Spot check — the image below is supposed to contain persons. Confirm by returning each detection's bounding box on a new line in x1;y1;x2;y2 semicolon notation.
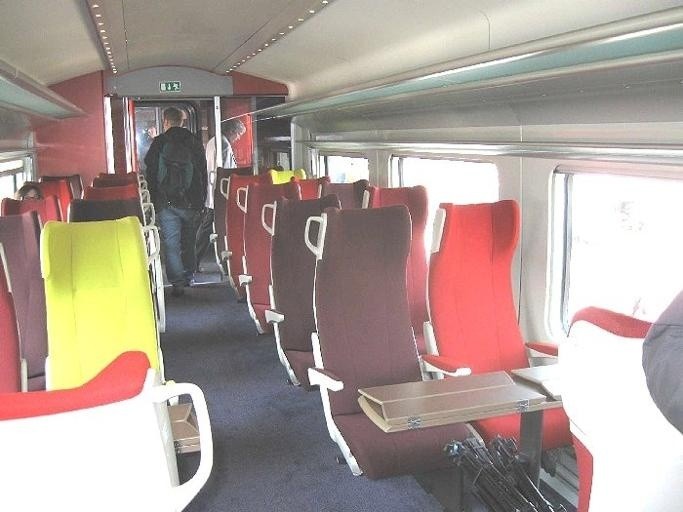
142;106;210;298
12;183;41;201
192;117;247;276
641;289;681;438
141;126;158;148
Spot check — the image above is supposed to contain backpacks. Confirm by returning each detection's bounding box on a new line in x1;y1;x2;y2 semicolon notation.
155;131;196;198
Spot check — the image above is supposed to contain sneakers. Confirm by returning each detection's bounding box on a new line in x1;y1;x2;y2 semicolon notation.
184;278;196;288
170;280;185;298
